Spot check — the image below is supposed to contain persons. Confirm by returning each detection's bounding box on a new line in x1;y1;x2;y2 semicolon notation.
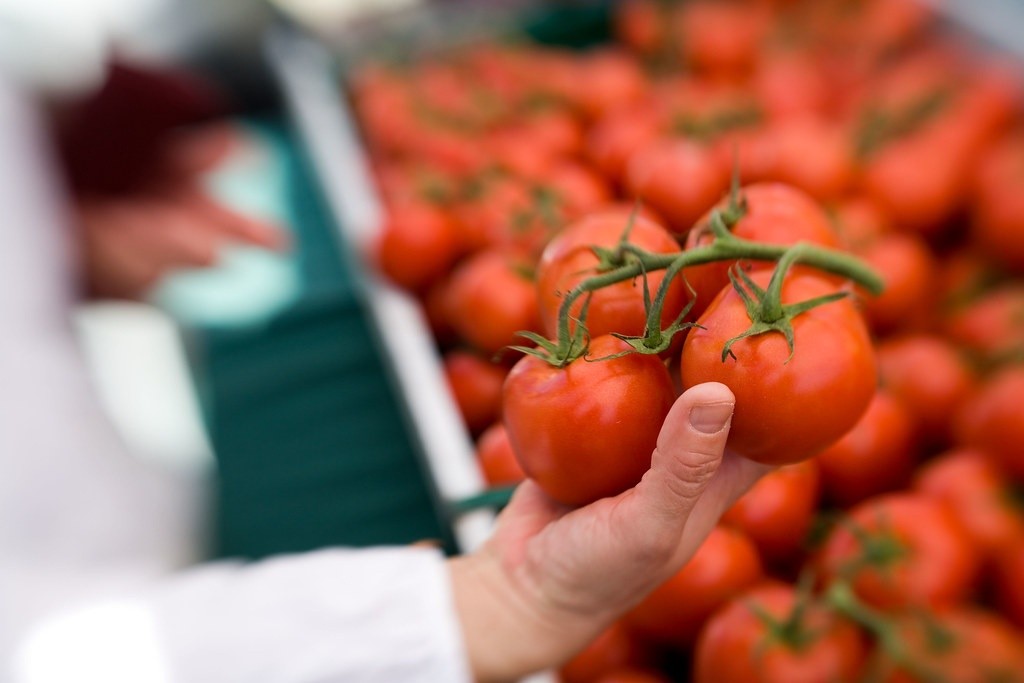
1;1;782;683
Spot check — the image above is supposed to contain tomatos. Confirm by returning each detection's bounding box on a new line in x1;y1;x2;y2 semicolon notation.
352;0;1024;683
